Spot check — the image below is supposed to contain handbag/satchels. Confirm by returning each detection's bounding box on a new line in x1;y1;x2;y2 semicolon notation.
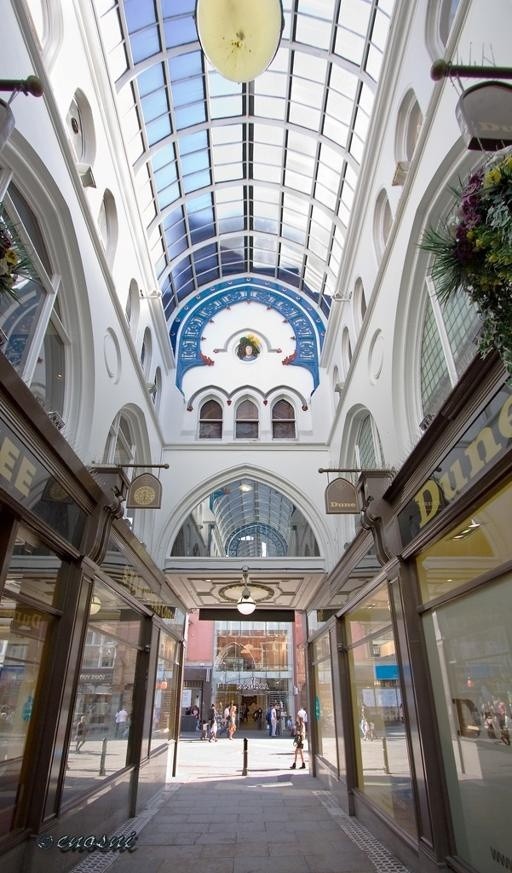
74;736;82;742
226;716;230;721
294;736;301;744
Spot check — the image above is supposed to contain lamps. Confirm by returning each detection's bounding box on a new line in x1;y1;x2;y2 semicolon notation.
236;567;256;616
195;0;283;85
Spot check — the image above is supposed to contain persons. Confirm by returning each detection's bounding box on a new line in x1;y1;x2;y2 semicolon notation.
359;702;375;741
267;702;281;736
290;716;306;770
493;695;511;745
115;706;129;739
200;702;238;743
185;705;199;731
74;715;88;754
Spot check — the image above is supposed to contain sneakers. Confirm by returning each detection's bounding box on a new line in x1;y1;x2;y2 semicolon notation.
299;764;306;769
290;763;296;769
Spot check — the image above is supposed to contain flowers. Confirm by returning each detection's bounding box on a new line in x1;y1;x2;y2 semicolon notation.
407;140;512;392
2;204;41;311
